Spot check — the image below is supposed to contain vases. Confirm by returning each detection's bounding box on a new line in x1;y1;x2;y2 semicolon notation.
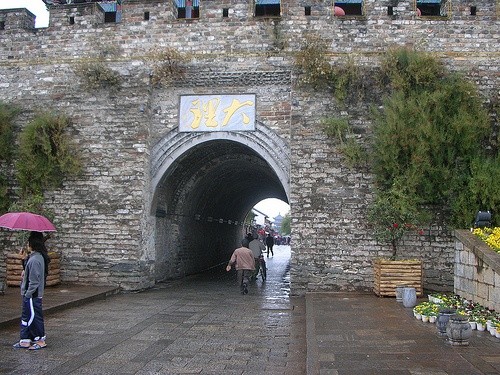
372;260;424;298
469;322;476;330
477;323;486;331
428;316;436;323
422;314;428;322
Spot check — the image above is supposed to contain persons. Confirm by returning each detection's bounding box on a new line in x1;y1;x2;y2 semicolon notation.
13;236;47;349
22;231;49;344
228;232;291;294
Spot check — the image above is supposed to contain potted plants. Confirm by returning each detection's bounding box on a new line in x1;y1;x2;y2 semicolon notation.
6;195;61;288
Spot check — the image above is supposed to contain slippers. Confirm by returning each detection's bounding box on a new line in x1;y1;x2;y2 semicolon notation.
28;343;47;350
13;342;32;348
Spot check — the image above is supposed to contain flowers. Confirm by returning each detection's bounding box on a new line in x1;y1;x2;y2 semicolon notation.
413;290;500;333
369;175;432;262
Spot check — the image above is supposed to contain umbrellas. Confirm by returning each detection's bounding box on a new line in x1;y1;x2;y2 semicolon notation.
0;212;57;248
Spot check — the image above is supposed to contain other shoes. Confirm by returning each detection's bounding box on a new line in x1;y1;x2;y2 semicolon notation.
241;291;246;295
243;285;248;293
